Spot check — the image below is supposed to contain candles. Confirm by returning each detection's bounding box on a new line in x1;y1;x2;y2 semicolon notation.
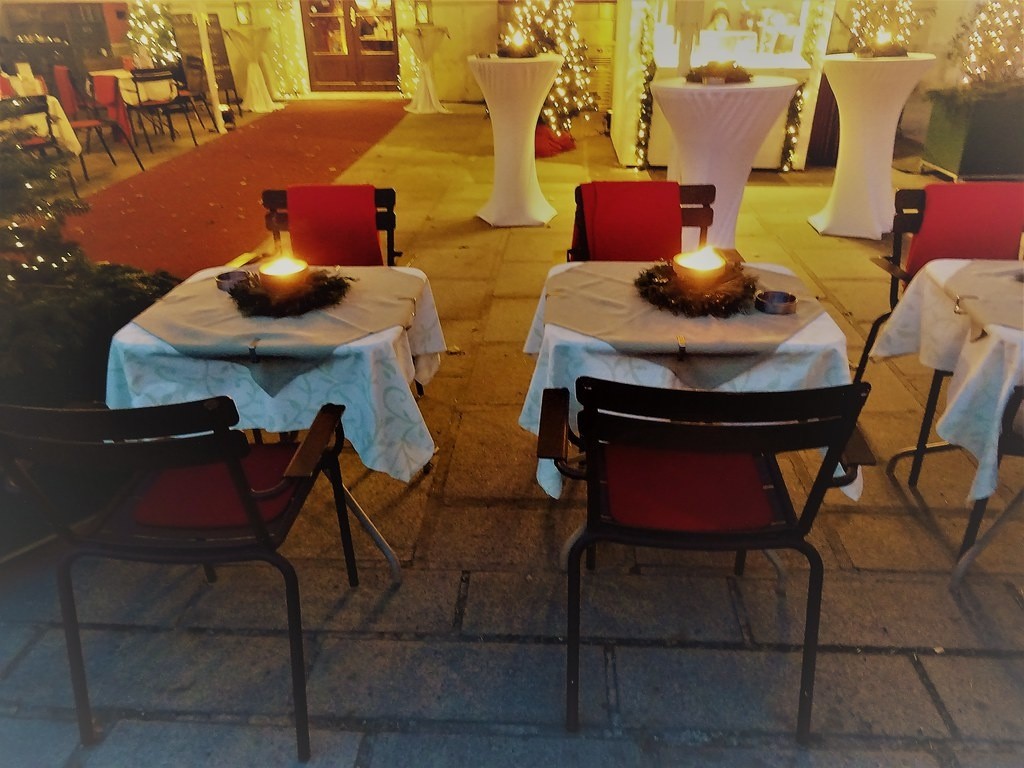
259;256;307;291
674;245;726;283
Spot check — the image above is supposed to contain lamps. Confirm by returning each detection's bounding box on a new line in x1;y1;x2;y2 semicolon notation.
235;1;251;26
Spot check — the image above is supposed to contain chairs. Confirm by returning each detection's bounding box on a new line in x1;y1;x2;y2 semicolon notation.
0;57;244;198
0;179;1024;745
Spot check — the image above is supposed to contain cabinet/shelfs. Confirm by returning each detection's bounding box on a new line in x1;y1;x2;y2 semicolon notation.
921;92;1024;183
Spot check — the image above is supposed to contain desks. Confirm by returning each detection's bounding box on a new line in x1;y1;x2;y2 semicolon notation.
867;258;1024;563
415;1;434;26
1;95;82;156
106;266;449;587
1;76;43;100
224;25;285;113
517;262;862;575
651;76;800;249
397;25;455;113
806;49;936;240
85;69;180;138
467;56;562;226
